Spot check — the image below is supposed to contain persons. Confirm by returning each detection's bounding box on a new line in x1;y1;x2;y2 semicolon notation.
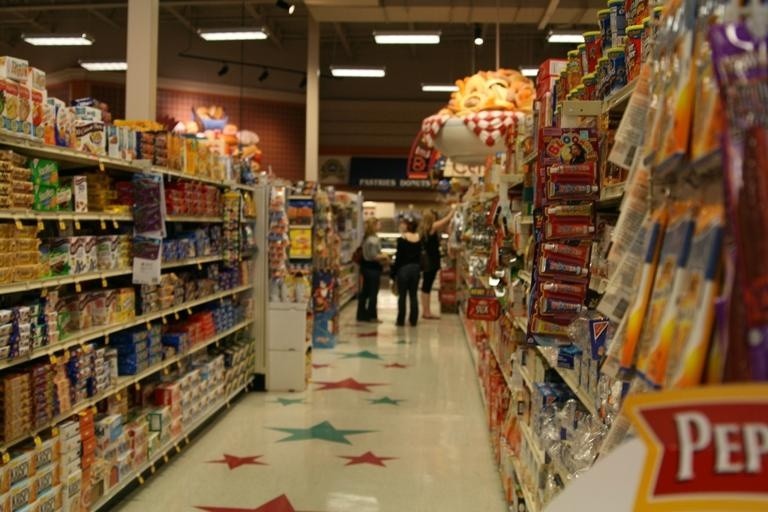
421;205;456;320
357;217;390;322
391;212;421;327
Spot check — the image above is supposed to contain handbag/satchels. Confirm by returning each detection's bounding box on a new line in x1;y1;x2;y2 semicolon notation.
354;235;366;262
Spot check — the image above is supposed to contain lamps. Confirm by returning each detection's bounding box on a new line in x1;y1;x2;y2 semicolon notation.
544;28;586;47
77;52;129;75
418;79;457;94
195;16;270;43
20;13;98;47
519;64;541;78
472;27;485;47
328;56;388;82
276;4;298;19
373;27;442;50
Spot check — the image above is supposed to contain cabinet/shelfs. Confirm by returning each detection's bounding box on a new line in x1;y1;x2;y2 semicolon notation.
0;131;269;512
